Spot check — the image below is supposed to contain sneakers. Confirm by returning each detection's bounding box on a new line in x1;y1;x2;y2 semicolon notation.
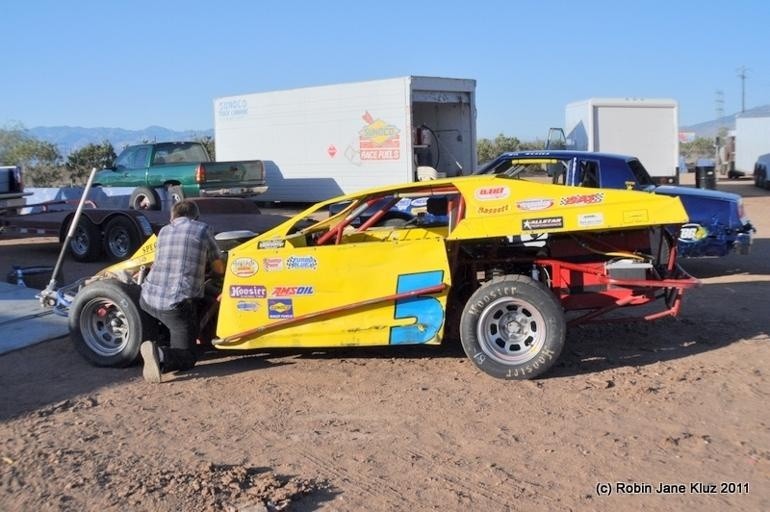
140;340;162;384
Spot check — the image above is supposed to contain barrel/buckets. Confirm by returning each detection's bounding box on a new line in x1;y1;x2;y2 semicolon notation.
695;166;717;190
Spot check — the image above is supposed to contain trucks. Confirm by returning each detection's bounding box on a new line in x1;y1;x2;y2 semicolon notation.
546;97;679;188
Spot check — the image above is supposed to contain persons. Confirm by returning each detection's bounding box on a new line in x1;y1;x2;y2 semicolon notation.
137;199;224;383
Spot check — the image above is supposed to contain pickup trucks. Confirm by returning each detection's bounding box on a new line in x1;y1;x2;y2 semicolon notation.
91;142;269;201
